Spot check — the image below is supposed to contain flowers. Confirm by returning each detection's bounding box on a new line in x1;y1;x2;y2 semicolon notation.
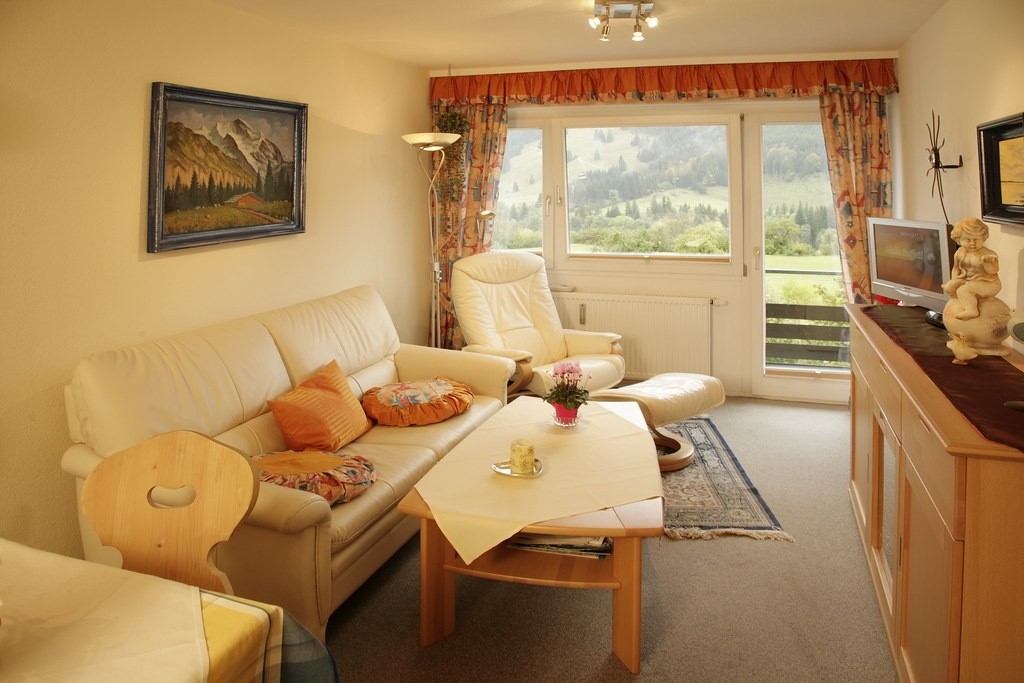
544;360;594;410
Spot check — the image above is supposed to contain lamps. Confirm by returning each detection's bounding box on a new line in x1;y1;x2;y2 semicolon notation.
432;210;496;255
589;0;658;42
402;132;461;350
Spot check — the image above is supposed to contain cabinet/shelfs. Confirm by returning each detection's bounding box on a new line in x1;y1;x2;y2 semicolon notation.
841;300;1024;683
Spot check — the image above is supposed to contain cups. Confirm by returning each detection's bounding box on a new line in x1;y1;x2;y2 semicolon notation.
510;438;534;474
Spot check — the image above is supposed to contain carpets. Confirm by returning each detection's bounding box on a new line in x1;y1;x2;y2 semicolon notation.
647;412;799;546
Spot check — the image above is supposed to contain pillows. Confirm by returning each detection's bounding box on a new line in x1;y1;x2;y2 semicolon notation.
362;375;473;428
248;446;375;510
266;359;375;453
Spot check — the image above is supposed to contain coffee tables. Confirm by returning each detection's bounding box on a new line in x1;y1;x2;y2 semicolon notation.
401;395;665;673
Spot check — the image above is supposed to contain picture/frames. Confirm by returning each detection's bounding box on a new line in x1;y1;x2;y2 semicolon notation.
144;81;309;252
975;111;1024;229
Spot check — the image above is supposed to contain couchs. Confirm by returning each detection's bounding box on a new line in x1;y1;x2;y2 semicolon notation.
60;283;519;651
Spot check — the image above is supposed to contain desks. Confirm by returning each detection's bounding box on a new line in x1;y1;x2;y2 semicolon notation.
0;535;337;683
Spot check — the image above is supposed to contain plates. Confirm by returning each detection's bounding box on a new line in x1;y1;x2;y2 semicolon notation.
491;458;544;478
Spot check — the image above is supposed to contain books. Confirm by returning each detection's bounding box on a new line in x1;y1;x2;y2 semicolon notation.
507;532;612;560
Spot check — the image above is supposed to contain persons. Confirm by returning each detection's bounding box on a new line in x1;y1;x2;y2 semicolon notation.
941;216;1002;320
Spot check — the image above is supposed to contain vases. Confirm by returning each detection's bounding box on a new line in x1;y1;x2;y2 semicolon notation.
548;401;583;426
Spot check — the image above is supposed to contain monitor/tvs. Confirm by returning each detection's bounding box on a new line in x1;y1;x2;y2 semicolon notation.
867;217;959;314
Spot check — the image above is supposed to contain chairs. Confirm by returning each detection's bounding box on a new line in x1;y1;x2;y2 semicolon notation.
75;429;262;597
449;249;725;473
